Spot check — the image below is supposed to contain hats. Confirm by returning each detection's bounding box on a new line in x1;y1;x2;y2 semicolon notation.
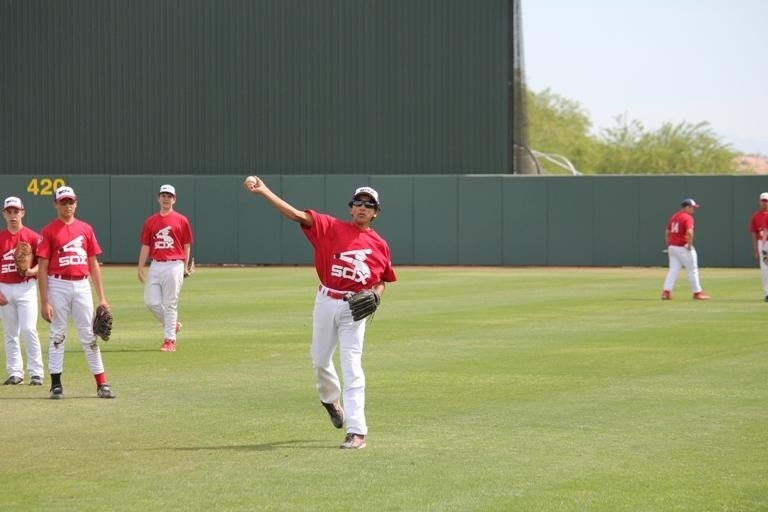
56;186;76;201
4;197;24;210
679;199;700;208
353;187;380;205
760;193;768;201
158;185;176;196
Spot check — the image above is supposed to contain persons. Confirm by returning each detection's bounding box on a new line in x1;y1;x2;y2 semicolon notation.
39;185;118;399
749;191;768;304
0;195;44;388
242;174;397;451
136;183;194;351
655;197;710;301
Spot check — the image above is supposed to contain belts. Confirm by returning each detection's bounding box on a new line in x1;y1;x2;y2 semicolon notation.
49;273;90;280
319;285;347;299
150;258;186;262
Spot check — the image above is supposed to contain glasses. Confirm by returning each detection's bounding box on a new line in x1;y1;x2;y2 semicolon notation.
352;199;376;208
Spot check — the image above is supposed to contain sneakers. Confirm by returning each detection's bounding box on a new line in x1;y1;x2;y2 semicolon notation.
320;399;345;428
693;293;710;299
160;339;176;352
30;375;43;384
50;385;64;399
176;322;181;333
340;433;366;449
3;376;23;385
97;384;115;398
661;291;670;300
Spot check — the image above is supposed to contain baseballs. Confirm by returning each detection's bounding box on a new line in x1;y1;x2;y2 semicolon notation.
246;177;256;186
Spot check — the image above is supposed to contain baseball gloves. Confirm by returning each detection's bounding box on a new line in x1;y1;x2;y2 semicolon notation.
13;239;33;277
94;306;113;342
184;256;195;278
344;289;380;321
762;251;768;265
